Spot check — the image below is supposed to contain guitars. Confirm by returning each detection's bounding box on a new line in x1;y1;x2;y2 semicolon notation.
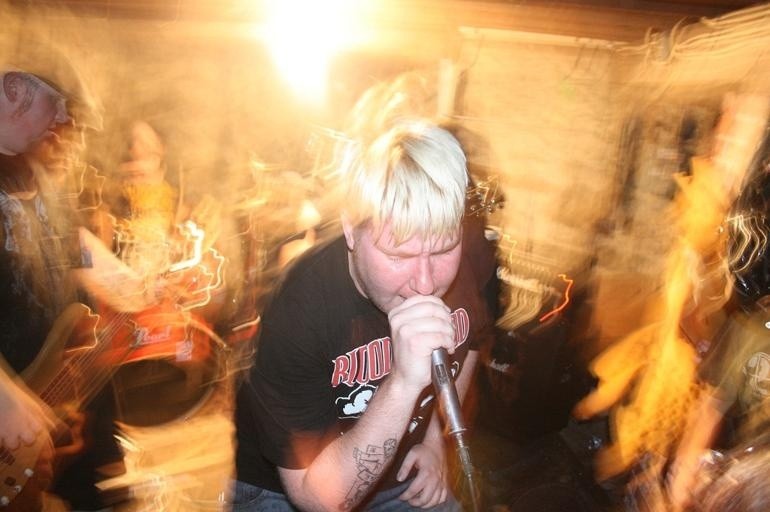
1;251;196;510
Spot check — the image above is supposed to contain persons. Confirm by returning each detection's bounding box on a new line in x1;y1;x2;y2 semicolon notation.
1;72;152;510
228;123;497;511
659;127;769;511
131;120;173;280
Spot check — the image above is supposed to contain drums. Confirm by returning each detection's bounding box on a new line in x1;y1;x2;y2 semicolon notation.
86;301;217;428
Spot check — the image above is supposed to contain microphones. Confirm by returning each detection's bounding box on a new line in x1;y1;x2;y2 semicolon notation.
431;349;469;454
